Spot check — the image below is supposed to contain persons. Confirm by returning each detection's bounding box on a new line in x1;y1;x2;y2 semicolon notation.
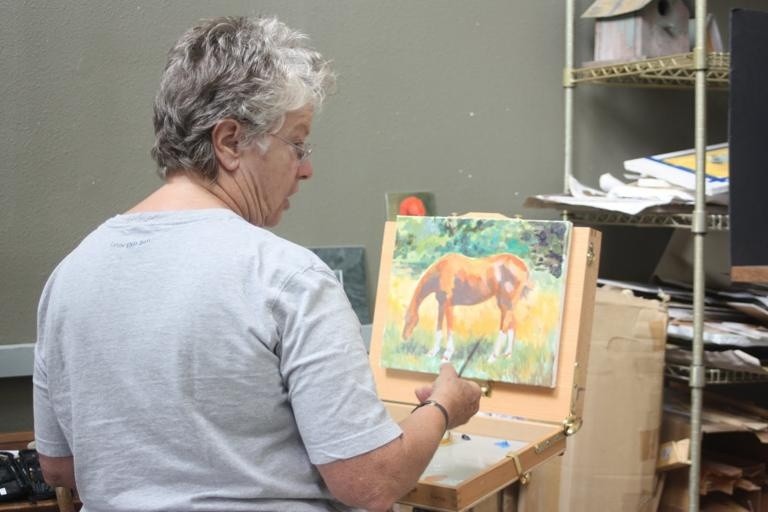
31;10;480;512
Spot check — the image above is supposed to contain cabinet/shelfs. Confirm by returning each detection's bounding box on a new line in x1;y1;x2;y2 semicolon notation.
561;0;766;512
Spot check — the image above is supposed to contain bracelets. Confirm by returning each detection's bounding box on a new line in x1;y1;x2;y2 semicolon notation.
408;400;451;433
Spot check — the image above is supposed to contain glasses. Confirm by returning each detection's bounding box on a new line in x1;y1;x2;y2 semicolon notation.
262;125;314;164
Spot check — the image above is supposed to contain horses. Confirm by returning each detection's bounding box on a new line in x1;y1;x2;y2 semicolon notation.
400;252;535;364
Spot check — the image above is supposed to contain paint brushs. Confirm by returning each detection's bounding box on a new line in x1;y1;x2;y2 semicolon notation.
457;333;485;379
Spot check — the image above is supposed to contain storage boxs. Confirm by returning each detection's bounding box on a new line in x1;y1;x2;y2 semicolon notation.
468;288;673;512
